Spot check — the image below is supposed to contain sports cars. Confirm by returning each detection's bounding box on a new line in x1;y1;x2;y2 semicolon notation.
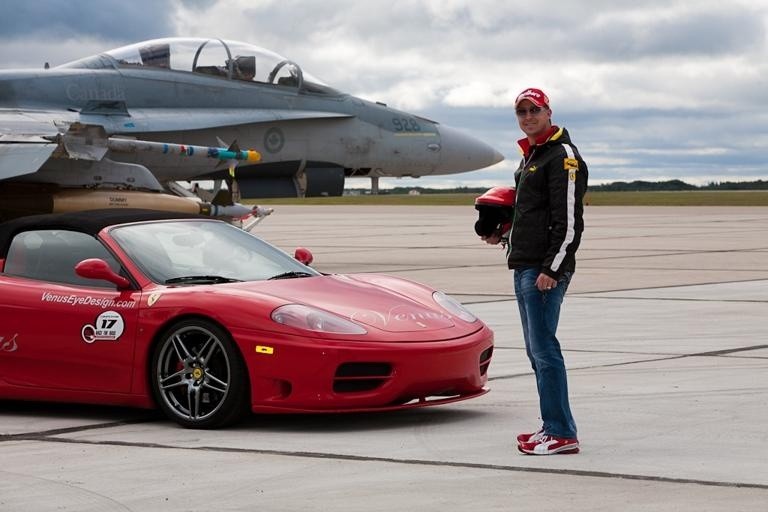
0;219;495;430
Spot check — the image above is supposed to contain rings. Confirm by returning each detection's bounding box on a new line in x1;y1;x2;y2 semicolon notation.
547;287;552;289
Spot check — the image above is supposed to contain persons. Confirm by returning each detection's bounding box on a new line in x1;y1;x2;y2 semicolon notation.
481;87;588;456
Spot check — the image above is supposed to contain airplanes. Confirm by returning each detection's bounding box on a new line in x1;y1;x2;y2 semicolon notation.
0;36;508;231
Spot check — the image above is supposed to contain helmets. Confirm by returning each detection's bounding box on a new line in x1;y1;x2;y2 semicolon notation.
474;186;516;238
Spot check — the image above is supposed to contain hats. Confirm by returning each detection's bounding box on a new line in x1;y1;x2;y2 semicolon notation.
515;88;549;109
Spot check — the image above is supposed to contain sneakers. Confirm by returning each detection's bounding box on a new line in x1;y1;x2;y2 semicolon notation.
517;427;580;455
